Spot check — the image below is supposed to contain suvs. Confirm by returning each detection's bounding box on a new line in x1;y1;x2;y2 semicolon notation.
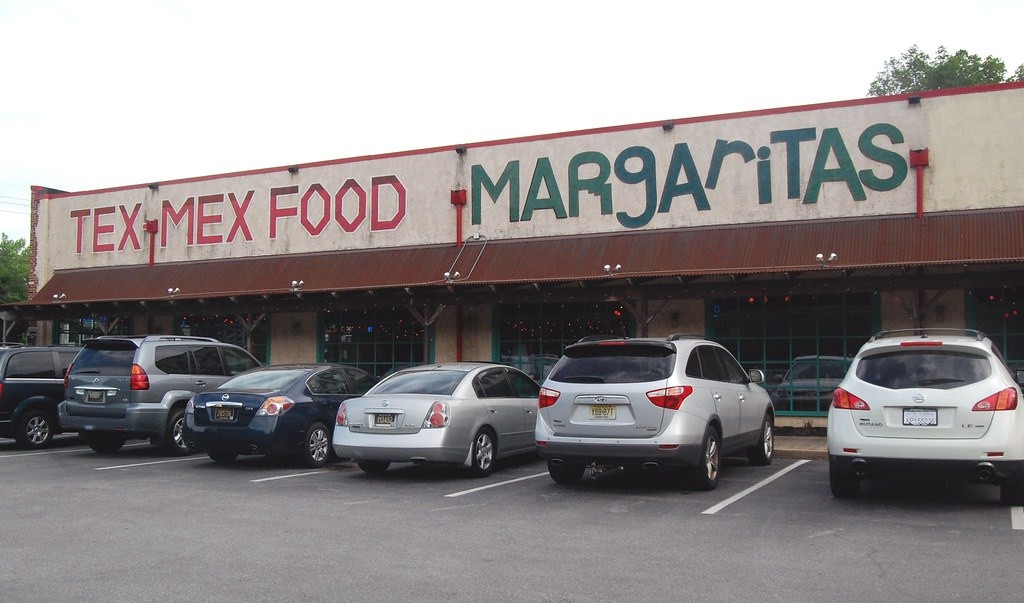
771;354;853;415
534;332;776;490
0;342;83;451
56;335;264;457
501;354;560;383
826;326;1023;499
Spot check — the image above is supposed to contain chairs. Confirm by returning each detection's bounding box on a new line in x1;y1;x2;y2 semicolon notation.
877;361;909;389
316;375;340;394
950;359;974;380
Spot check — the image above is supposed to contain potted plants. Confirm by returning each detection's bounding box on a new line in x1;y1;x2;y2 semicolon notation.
51;294;66;302
289;280;305;290
167;288;181;297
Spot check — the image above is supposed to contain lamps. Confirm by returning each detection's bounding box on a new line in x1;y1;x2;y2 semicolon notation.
148;184;159;190
934;305;945;322
815;252;837;269
443;272;460;282
287;168;298;173
663;123;674;129
455;147;467;153
604;264;622;278
907;95;922;104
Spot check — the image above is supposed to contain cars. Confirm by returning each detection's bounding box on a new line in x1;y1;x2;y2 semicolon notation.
332;363;540;477
182;364;379;468
